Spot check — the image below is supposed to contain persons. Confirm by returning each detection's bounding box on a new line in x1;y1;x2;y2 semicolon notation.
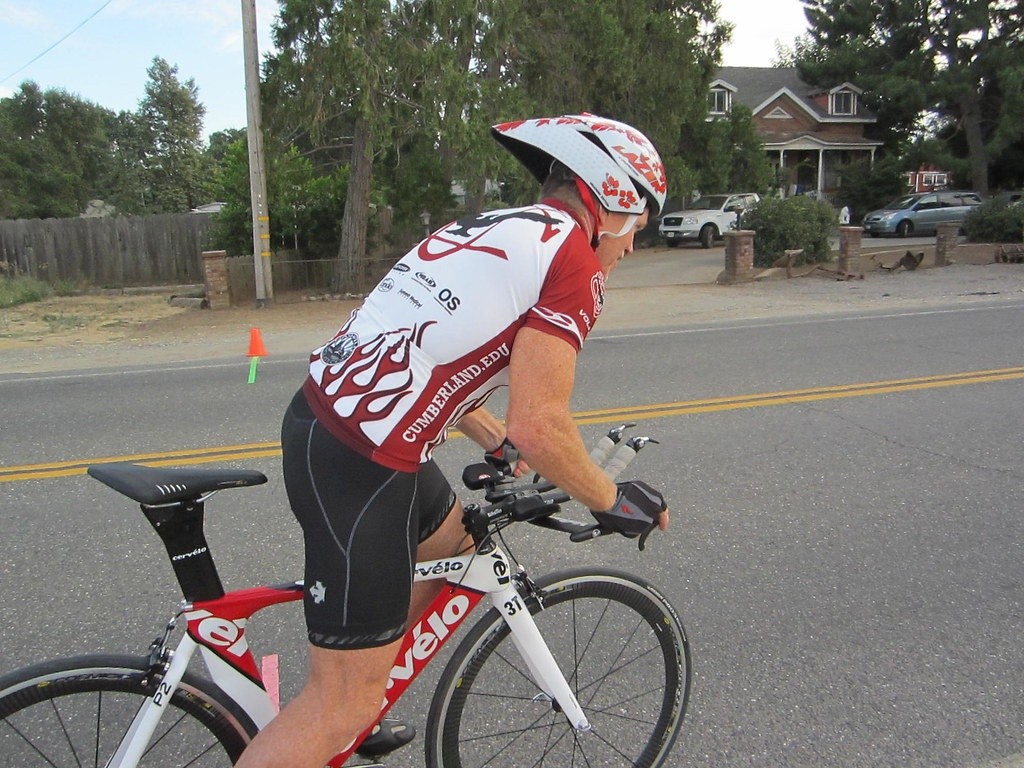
231;112;673;768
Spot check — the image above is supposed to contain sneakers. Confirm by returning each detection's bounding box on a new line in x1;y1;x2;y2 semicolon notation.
353;718;415;756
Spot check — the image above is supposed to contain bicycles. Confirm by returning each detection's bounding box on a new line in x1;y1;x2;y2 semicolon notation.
0;422;692;768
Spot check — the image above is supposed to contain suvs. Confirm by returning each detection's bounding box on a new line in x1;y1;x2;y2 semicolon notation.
659;192;760;249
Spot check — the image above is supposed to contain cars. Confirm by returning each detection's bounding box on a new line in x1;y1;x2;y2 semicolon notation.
864;191;984;238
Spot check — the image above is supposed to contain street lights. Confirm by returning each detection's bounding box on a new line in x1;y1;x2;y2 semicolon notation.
419;207;430;238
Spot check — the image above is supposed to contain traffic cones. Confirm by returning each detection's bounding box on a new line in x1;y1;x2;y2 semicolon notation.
246;327;267;357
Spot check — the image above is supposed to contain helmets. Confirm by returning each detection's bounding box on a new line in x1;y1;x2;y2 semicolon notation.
491;110;667;219
